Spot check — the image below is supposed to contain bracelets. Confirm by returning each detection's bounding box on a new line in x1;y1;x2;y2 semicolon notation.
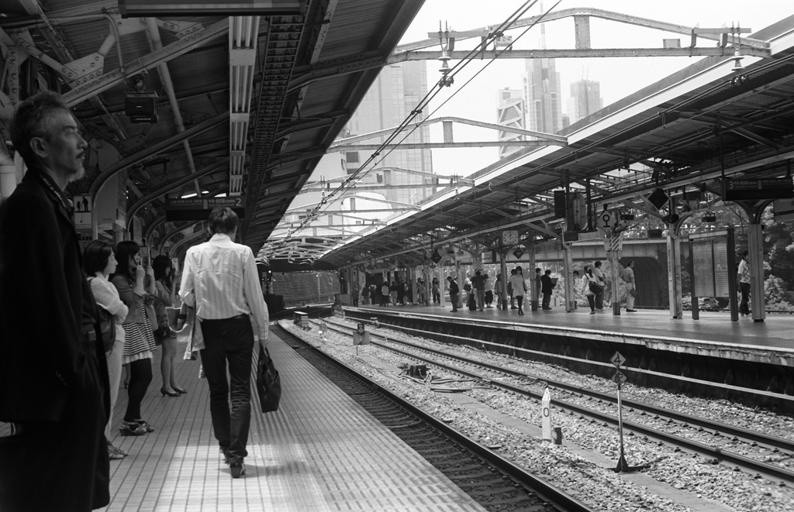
176;312;186;320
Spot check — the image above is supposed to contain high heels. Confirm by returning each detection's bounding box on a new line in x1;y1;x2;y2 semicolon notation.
161;387;180;397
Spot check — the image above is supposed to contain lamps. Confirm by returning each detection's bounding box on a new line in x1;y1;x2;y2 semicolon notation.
228;15;260;197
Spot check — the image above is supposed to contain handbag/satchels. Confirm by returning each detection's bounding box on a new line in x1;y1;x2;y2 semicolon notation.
153;324;170;341
256;339;281;413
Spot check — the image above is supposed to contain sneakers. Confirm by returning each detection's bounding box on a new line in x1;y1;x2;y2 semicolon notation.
107;440;128;458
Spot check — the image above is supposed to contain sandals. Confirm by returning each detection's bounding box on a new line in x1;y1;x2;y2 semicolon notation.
119;421;147;436
135;419;154;433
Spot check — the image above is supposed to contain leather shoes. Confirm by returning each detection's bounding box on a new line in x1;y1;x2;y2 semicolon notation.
171;384;186;393
232;463;246;478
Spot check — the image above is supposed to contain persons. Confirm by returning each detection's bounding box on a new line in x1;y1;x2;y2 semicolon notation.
80;239;127;460
0;90;110;512
360;258;637;316
738;250;751;315
176;206;269;478
108;241;157;435
146;255;187;397
